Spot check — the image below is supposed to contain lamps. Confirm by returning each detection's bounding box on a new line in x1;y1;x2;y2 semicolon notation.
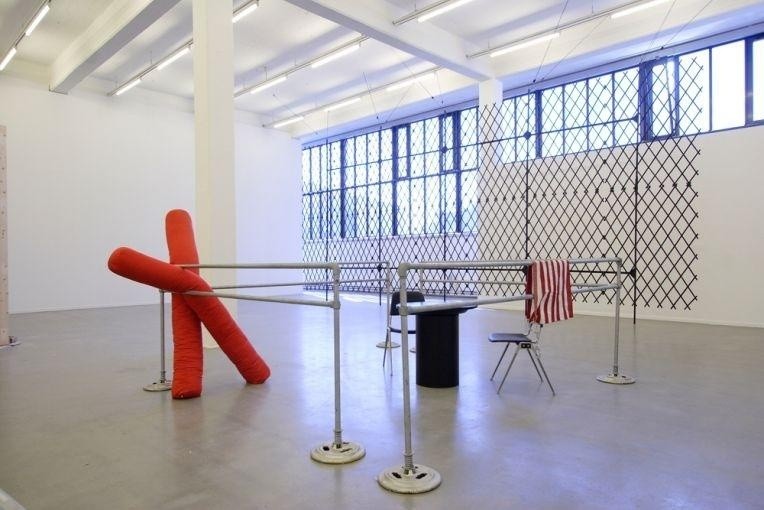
462;0;672;60
0;0;52;72
233;0;259;23
112;39;193;95
262;66;445;128
231;37;369;98
392;0;478;27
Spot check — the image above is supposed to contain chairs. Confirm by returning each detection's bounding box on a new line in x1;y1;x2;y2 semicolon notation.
383;290;425;376
488;322;555;397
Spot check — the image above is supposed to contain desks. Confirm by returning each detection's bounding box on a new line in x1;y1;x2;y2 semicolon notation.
410;307;478;389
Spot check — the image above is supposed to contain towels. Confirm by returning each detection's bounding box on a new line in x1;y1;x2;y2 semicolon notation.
523;259;574;323
